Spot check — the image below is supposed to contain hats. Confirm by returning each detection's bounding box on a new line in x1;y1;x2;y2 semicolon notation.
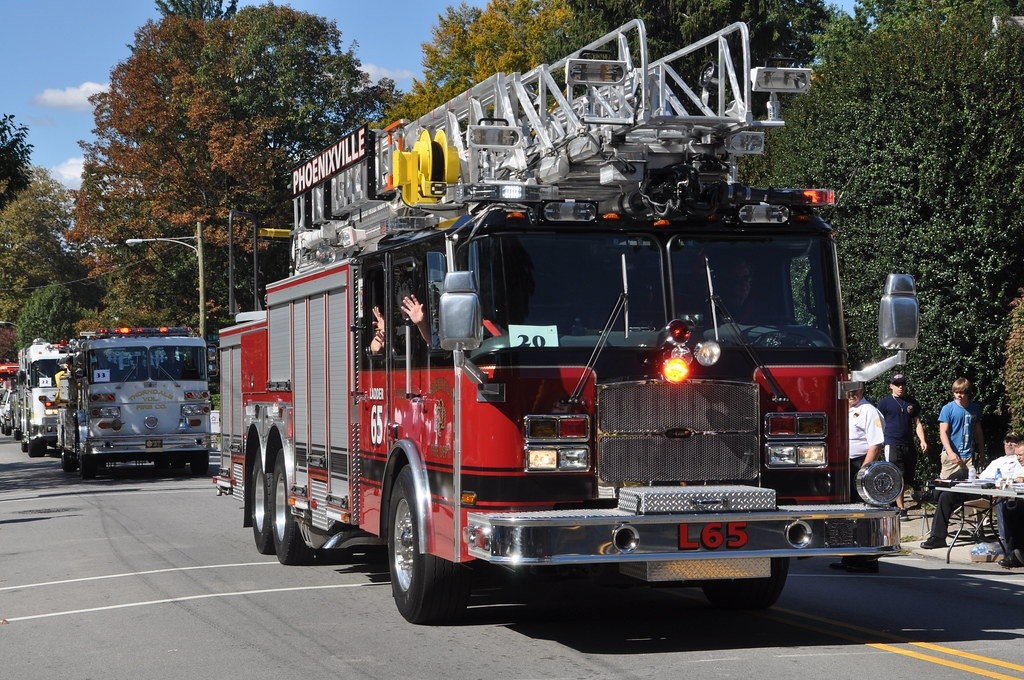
1005;431;1019;441
891;374;905;384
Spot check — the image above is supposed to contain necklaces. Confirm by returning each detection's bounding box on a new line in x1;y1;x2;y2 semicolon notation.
893;396;905;412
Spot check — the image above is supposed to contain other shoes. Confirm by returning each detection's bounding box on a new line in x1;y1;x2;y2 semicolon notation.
999;549;1024;566
911;489;934;503
921;537;946;548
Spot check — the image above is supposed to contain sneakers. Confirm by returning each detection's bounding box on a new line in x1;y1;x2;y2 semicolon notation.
899;508;908;521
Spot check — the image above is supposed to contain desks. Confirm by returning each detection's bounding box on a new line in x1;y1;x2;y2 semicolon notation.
936;487;1024;566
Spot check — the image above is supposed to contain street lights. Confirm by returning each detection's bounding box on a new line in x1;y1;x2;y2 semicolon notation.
124;221;206;340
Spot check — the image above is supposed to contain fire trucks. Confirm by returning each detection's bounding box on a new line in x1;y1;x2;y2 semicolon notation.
209;15;921;630
0;326;218;479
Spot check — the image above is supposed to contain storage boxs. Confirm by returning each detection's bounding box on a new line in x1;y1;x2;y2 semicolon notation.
971;554;992;562
991;554;1005;562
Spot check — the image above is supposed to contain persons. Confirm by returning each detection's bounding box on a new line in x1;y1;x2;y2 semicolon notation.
829;381;886;573
940;378;995;528
920;432;1024;568
369;282;414;356
400;294;431;344
877;374;928;522
